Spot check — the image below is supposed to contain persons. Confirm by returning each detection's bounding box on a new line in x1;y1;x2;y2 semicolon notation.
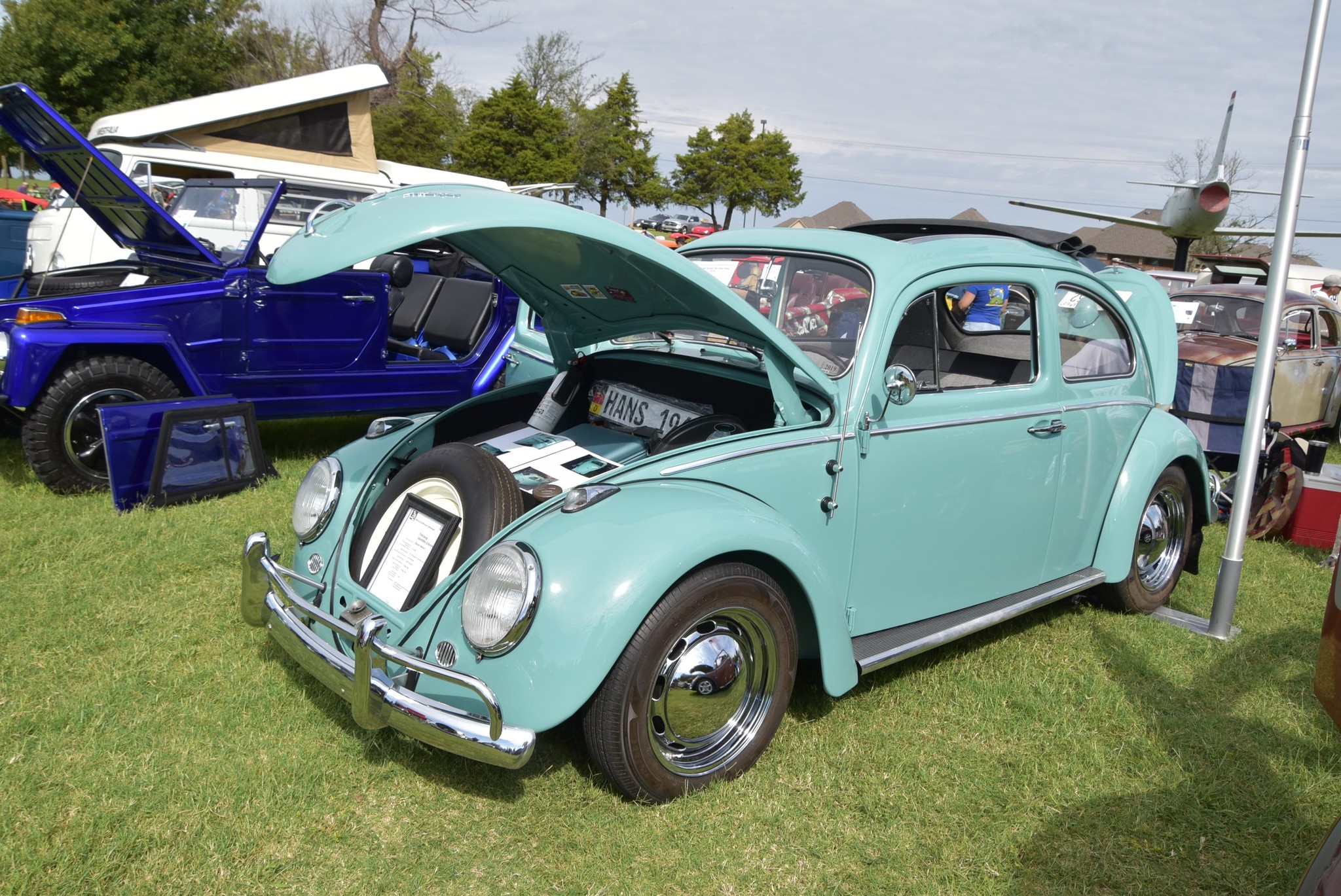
28;183;39;192
958;283;1010;332
1310;274;1341;299
17;182;29;194
642;231;647;236
676;236;685;247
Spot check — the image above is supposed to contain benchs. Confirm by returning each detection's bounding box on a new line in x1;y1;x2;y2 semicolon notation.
1238;331;1335;347
885;342;1103;385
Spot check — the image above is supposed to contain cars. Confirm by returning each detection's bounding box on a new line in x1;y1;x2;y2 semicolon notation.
237;185;1222;807
1168;283;1341;456
1144;254;1341;320
633;215;871;339
0;81;519;512
128;177;378;280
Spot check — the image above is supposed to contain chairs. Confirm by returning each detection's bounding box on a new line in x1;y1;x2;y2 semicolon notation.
786;272;814;307
815;274;854;302
801;350;853;376
1294;344;1312;350
386;278;494;363
910;368;1011;389
343;253;413;320
387;271;447;342
786;280;816;307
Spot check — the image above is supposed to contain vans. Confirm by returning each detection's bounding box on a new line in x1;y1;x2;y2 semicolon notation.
23;143;393;276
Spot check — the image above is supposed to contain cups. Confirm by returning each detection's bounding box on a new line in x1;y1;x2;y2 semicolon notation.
1304;440;1329;476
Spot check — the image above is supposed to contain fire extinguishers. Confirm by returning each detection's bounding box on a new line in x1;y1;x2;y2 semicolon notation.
528;351;586;433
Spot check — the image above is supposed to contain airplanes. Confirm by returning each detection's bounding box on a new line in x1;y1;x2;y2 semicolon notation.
1008;90;1341;246
